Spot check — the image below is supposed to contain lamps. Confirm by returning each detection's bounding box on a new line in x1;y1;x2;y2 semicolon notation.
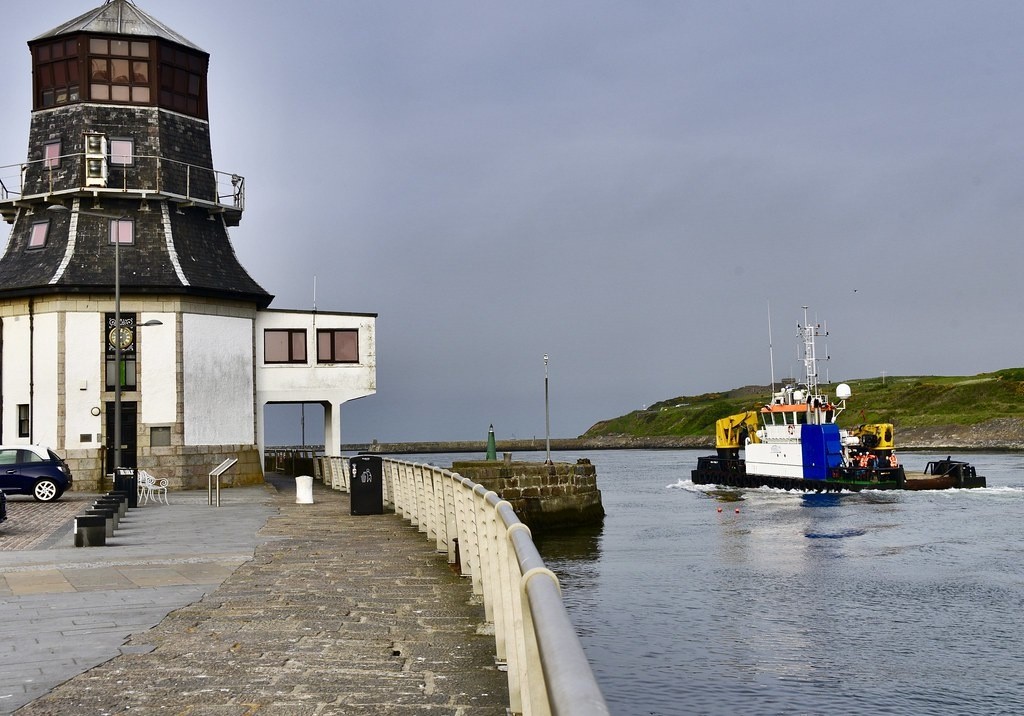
175;208;184;215
138;202;152;212
90;202;104;210
25;209;34;216
207;215;215;221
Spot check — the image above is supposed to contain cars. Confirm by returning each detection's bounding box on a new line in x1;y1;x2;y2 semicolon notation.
0;443;74;503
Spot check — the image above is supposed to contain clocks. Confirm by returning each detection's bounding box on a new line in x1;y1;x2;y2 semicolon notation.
111;328;131;346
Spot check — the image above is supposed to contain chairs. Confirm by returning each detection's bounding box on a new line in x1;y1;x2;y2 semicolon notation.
138;470;169;505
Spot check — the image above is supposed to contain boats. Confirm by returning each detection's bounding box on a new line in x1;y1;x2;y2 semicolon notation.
689;298;988;496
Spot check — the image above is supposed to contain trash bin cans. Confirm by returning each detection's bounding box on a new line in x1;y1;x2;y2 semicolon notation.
351;456;382;514
113;469;138;508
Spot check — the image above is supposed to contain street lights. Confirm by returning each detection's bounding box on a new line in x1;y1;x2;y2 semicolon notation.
46;203;164;485
